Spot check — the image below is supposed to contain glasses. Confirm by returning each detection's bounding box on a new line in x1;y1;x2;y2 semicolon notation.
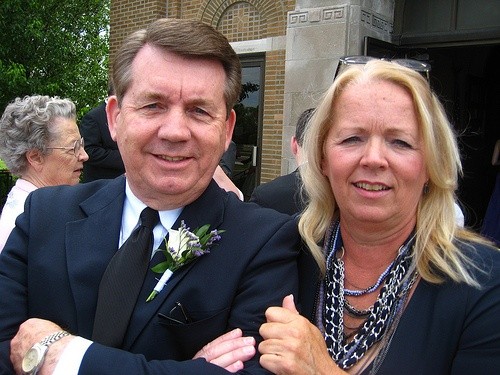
46;137;84;158
334;55;431;84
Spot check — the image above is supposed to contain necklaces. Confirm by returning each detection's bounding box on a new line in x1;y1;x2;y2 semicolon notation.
310;207;421;375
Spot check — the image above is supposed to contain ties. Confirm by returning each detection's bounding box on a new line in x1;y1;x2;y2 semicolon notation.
93;206;160;349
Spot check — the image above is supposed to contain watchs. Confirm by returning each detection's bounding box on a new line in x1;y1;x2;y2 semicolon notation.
21;331;71;374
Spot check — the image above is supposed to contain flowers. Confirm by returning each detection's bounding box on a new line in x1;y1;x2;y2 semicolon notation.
146;220;227;301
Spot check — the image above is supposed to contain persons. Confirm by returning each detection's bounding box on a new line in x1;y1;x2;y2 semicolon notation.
78;86;126;183
258;56;500;375
1;94;89;255
0;18;301;375
249;108;315;216
